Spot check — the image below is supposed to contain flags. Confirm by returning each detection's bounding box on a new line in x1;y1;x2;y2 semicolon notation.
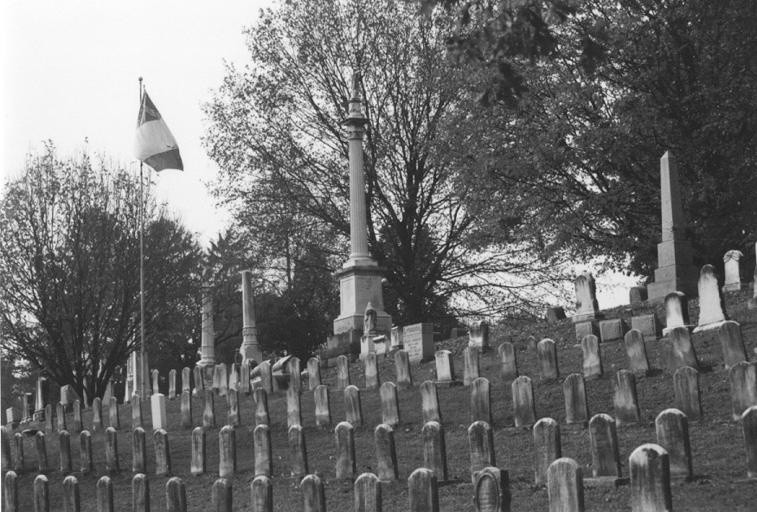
133;87;184;172
